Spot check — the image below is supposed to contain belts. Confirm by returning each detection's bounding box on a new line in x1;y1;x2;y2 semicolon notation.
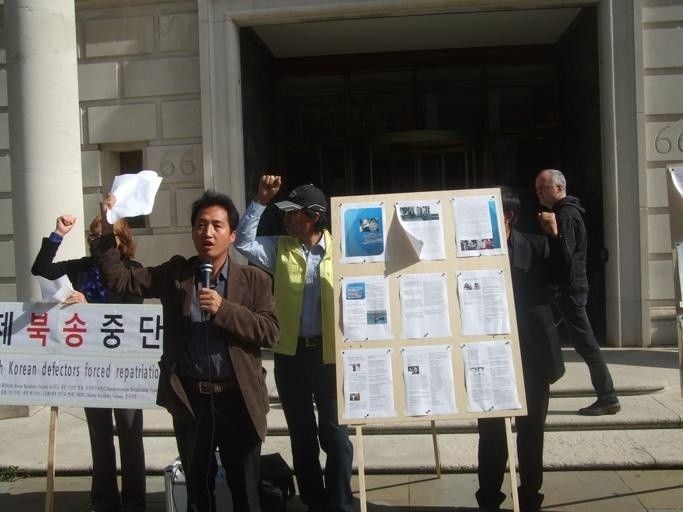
194;382;233;394
299;336;322;347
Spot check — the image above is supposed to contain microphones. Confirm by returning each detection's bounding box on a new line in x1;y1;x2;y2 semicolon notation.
198;255;213;316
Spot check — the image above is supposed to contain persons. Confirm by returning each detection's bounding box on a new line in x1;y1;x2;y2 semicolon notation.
535;168;621;417
474;185;575;512
232;173;354;512
30;213;146;511
88;188;282;512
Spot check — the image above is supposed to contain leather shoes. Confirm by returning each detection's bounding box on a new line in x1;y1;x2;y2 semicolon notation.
579;400;621;416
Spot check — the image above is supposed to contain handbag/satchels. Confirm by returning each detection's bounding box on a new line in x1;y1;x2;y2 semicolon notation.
258;453;295;512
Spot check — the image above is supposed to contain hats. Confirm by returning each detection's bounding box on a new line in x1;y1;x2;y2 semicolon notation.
274;184;327;212
